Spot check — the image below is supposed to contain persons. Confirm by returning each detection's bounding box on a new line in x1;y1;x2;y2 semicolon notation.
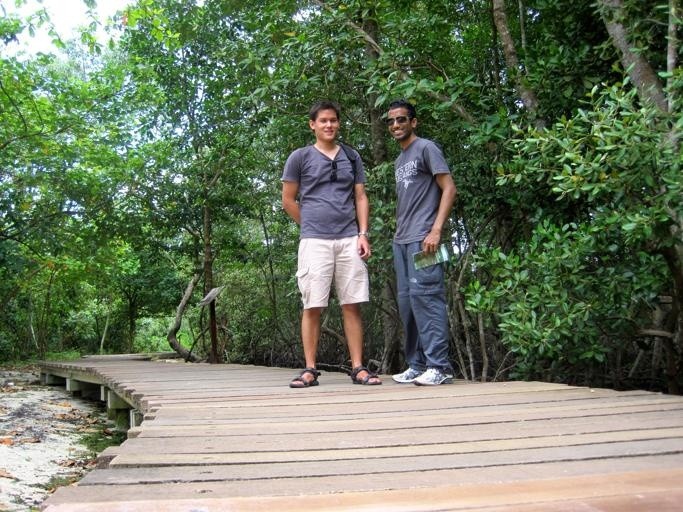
386;99;457;386
279;101;381;388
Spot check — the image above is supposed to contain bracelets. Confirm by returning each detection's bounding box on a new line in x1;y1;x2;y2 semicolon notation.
359;232;369;237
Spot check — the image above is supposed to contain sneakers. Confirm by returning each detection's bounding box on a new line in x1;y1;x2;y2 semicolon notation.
391;367;423;383
414;368;454;385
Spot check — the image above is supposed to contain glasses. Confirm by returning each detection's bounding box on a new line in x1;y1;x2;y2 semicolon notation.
330;160;338;182
386;116;410;125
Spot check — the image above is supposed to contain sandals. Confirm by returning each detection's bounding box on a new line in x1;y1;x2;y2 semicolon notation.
289;368;318;387
350;367;381;384
